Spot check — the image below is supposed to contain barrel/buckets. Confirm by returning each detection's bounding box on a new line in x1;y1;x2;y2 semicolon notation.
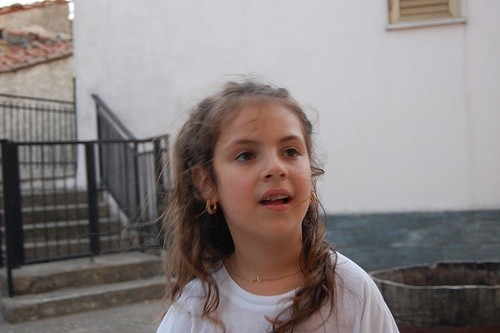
366;259;500;333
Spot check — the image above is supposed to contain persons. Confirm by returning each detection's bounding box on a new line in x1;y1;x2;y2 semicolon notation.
155;80;399;333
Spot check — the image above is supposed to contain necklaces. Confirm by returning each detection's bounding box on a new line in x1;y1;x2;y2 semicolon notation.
227;254;306;283
